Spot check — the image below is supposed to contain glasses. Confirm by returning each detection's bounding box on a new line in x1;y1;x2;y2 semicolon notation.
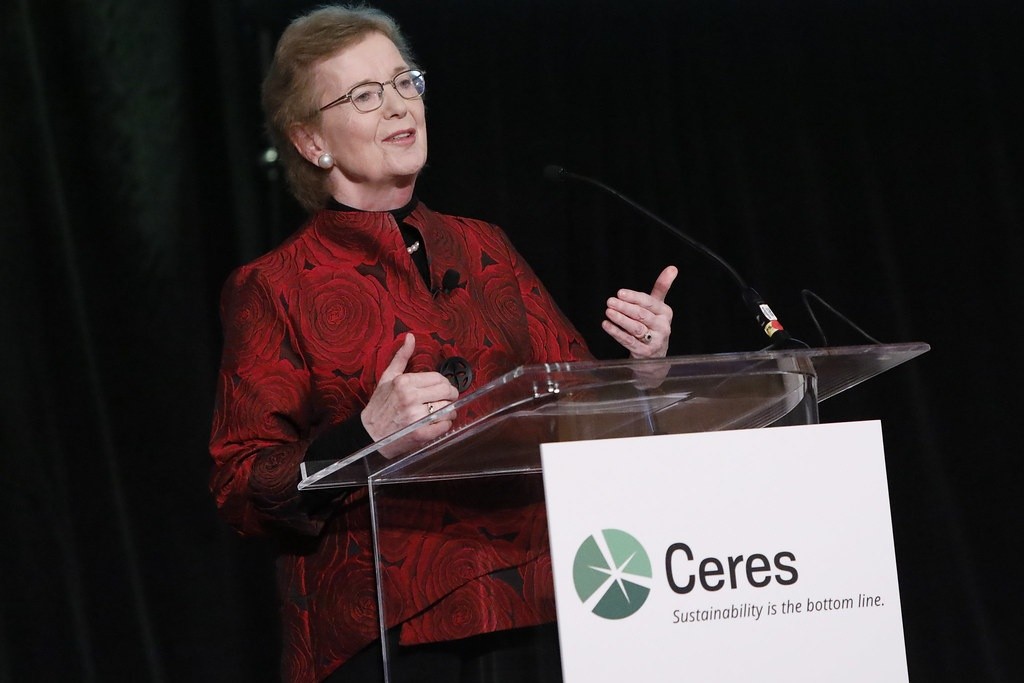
303;69;427;118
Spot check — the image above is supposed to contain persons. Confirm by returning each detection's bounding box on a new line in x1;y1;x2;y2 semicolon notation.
211;4;679;683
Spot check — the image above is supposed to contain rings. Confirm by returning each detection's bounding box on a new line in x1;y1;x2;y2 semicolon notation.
640;329;653;343
427;402;434;414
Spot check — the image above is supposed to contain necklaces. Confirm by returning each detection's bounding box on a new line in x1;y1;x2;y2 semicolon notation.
407;240;420;254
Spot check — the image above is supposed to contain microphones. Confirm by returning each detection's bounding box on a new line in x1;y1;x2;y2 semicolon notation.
431;269;460;300
544;164;808;350
